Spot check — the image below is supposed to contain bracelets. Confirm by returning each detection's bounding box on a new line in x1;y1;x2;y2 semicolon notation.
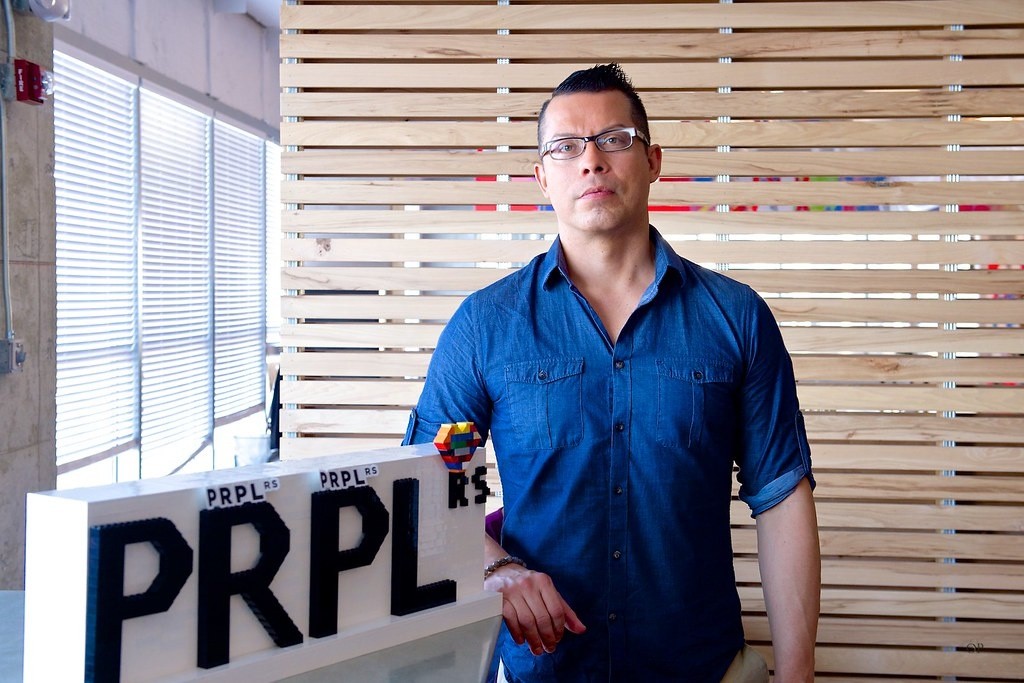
484;556;527;581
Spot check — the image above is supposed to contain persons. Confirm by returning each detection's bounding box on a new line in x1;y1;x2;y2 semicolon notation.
400;63;821;683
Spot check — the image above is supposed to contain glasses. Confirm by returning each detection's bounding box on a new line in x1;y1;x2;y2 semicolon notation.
539;127;649;160
